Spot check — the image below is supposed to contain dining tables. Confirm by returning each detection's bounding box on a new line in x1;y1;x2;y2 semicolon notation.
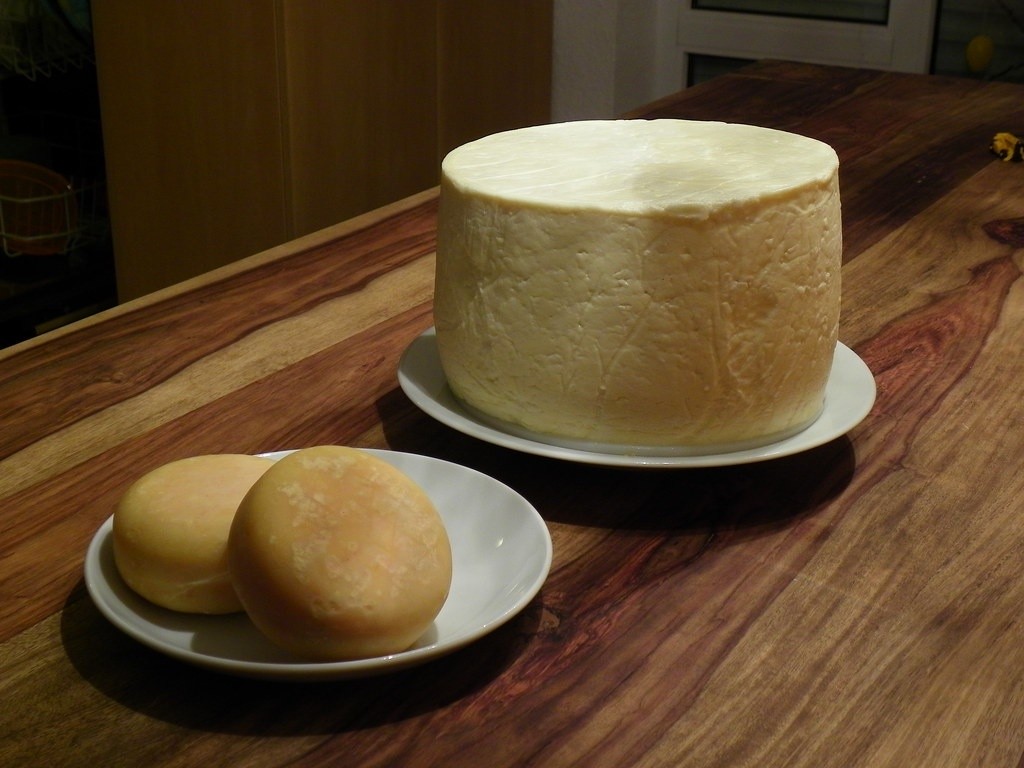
1;67;1024;768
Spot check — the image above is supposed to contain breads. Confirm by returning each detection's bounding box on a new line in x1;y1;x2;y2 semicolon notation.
433;116;843;448
224;445;453;662
112;455;282;616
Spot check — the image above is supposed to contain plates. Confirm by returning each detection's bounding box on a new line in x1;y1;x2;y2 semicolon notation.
84;448;553;685
397;323;877;468
